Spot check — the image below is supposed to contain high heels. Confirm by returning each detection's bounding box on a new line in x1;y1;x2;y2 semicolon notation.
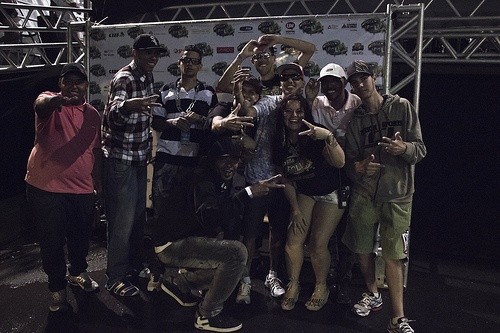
304;286;330;311
281;278;302;310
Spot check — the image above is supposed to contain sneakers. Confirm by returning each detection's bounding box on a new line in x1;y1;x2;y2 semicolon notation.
353;292;383;316
161;281;198;307
236;279;251;304
146;272;164;292
104;272;139;296
386;316;416;333
265;275;285;298
194;310;242;332
178;268;203;295
126;267;151;279
48;289;64;312
68;271;99;292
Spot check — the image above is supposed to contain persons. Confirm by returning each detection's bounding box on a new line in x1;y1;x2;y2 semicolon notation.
311;62;361;305
150;45;219;223
209;61;306;304
271;94;352;310
152;137;286;332
335;60;427;333
215;33;316;96
24;63;102;313
100;33;165;296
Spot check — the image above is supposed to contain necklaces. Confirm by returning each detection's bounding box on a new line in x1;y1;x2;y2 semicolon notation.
175;83;198;113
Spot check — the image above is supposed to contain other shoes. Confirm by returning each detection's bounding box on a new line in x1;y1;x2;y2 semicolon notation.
328;269;340;293
338;277;352;305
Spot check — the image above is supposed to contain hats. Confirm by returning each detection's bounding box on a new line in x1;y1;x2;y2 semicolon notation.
317;63;347;82
133;34;161;50
208;136;243;158
60;63;87;81
275;63;305;80
346;60;372;83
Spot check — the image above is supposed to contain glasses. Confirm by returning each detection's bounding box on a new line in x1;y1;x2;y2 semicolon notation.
179;57;201;65
251;51;273;59
278;73;301;81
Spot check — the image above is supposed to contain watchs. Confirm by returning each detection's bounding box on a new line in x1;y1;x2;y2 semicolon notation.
326;132;334;143
